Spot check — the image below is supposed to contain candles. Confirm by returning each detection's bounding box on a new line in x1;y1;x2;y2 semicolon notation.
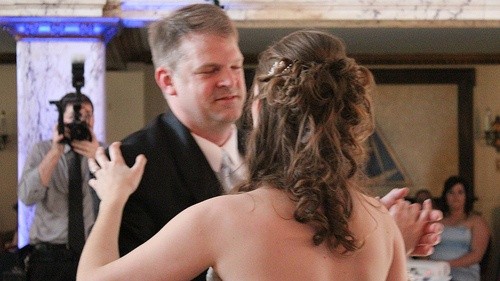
484;109;490;131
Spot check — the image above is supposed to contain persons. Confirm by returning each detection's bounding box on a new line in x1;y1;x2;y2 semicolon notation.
87;2;445;281
16;91;110;281
426;175;490;281
75;29;432;281
414;188;434;206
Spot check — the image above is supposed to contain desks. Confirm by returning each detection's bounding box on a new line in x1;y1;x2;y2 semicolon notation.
405;259;453;281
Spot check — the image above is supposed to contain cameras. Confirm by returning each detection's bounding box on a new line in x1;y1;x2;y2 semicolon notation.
58;56;93;143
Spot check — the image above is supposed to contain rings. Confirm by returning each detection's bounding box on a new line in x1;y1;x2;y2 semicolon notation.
91;167;101;175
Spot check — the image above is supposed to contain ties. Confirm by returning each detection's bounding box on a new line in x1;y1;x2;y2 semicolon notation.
68;150;87;258
218;149;245;195
